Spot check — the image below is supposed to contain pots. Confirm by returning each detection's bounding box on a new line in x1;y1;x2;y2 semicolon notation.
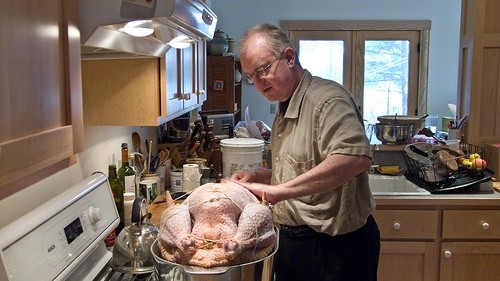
410;145;458;176
149;225;279;281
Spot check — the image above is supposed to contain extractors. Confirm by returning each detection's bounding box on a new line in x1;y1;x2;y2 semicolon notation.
78;0;219;60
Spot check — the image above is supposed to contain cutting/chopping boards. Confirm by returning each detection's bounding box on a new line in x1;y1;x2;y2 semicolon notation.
147;195;185;229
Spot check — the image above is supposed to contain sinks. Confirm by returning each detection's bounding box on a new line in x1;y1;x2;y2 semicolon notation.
368;178;420;193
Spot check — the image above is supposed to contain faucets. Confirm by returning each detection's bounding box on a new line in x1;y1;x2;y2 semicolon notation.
369;164;379;174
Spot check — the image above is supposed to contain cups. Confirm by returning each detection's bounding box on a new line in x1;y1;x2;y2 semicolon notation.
448;128;462;142
169;168;183;192
139;179;157;205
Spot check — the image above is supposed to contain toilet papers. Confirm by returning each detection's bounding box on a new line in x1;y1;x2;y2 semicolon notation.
182;164;199;194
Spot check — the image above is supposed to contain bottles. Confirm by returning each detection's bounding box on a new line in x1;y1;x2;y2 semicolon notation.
104;164;126;252
117;143;135;193
123;193;135;227
128;154;141;197
235;70;242;83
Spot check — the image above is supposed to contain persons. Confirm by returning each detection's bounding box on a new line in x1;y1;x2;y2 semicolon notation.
219;22;381;281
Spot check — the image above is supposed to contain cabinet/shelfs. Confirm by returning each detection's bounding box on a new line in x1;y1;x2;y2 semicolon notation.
81;41;208;127
372;196;500;281
202;56;242;126
0;0;84;187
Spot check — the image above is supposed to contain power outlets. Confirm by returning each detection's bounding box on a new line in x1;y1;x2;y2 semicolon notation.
269;103;278;115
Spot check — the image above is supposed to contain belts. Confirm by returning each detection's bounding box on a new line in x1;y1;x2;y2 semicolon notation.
279;224;320;239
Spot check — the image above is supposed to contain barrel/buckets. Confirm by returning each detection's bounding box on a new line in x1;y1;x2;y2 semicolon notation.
219;137;265;177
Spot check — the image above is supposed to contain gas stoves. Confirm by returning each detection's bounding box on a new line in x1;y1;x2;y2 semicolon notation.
0;171;153;281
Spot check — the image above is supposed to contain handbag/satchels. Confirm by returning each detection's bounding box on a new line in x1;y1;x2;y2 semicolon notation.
233;105;272;144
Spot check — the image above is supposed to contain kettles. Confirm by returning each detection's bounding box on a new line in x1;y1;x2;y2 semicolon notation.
112;196;159;274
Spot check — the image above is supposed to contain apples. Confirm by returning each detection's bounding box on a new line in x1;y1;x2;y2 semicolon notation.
458;153;487;170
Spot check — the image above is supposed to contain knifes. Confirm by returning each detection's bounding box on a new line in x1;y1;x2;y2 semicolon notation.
181;116;218;157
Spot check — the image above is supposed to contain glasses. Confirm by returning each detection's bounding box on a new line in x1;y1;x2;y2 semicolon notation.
245;51;284;85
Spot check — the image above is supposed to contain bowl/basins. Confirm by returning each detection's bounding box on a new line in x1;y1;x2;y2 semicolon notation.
377;115;426;138
375;123;413;146
404;142;434;161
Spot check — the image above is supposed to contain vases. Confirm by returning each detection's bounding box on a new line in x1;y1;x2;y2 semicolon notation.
235;69;242;83
